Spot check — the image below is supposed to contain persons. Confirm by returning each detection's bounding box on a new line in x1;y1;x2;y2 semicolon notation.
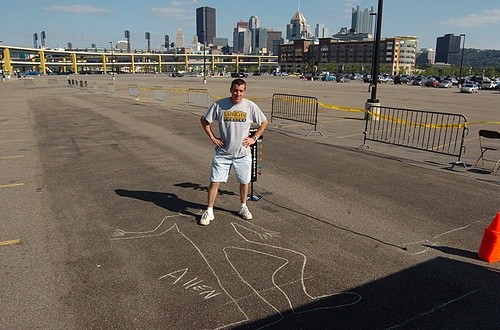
200;78;269;226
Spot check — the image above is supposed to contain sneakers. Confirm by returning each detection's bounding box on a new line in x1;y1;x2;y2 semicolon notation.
200;209;215;225
239;204;253;220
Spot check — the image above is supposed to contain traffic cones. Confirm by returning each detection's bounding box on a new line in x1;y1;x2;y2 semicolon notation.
478;212;500;263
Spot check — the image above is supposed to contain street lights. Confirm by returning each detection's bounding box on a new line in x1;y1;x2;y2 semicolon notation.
109;41;112;74
458;33;465;86
200;30;207;84
367;12;380;104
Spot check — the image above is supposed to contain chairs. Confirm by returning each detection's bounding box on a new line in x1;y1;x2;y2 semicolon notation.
474;129;500;175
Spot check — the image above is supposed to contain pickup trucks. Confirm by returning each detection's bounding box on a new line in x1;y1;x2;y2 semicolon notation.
231;69;249;78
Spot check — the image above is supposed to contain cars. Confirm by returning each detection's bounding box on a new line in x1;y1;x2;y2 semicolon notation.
299;70;500;90
273;71;287;76
460;84;478;94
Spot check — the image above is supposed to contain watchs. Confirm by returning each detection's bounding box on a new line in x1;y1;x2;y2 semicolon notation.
252;136;257;141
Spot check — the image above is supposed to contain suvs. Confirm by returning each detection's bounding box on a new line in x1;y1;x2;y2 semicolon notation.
172;72;183;77
